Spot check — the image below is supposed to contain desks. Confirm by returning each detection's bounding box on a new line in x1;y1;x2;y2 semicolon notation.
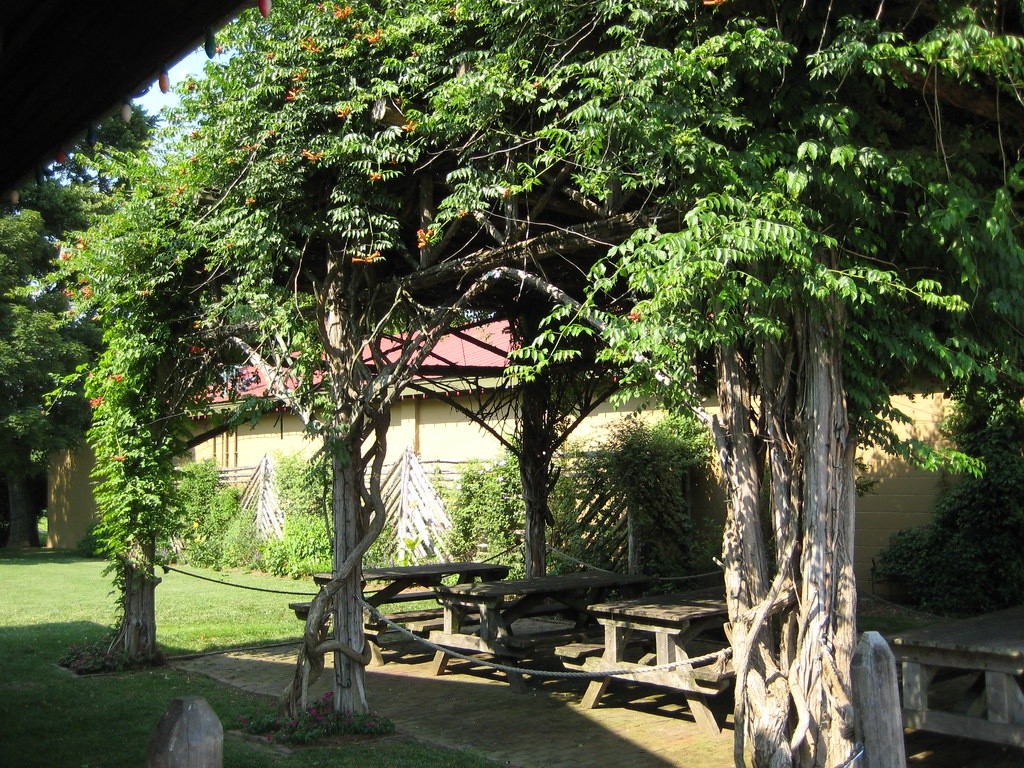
429;569;660;696
313;561;516;666
891;607;1024;748
579;586;729;735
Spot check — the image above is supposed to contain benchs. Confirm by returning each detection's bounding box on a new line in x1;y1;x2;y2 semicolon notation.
288;591;972;696
